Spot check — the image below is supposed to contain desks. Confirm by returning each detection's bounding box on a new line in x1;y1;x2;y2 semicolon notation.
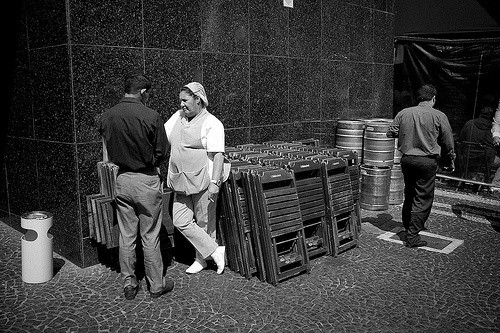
86;161;173;273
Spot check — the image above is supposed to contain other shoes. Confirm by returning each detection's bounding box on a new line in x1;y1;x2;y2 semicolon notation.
186;257;207;274
210;246;226;275
124;285;138;300
405;241;427;248
150;279;174;298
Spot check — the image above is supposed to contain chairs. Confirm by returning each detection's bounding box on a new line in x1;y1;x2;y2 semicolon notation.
217;138;361;286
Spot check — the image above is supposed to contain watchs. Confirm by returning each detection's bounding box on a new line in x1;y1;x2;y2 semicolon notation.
210;179;222;188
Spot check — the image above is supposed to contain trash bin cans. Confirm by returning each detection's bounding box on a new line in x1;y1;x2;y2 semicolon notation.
21;211;54;284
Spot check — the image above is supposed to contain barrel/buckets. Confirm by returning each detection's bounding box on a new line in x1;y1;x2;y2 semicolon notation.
335;118;404;211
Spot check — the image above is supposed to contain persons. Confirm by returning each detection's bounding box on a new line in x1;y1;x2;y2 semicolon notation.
164;82;232;274
459;104;498;192
97;71;174;300
386;84;456;248
489;99;500;201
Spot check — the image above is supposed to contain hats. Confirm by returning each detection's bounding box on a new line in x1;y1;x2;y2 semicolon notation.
184;81;208;107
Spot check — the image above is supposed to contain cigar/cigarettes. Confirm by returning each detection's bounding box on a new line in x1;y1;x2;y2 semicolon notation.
209;197;215;203
